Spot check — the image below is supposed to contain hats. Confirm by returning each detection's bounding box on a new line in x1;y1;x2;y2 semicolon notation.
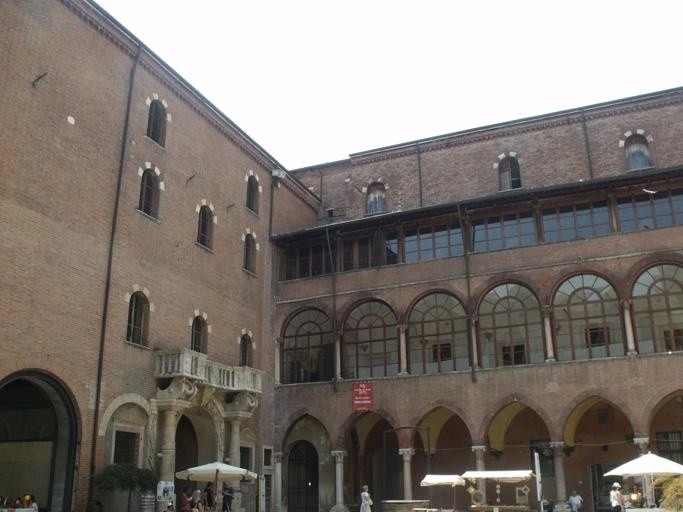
612;482;621;488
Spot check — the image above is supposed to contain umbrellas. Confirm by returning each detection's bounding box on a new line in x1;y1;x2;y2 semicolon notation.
176;461;257;496
603;451;683;503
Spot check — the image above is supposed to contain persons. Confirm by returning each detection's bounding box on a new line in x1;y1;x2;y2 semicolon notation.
178;487;204;512
566;490;584;512
360;485;373;512
222;481;234;512
610;482;643;512
204;482;214;507
